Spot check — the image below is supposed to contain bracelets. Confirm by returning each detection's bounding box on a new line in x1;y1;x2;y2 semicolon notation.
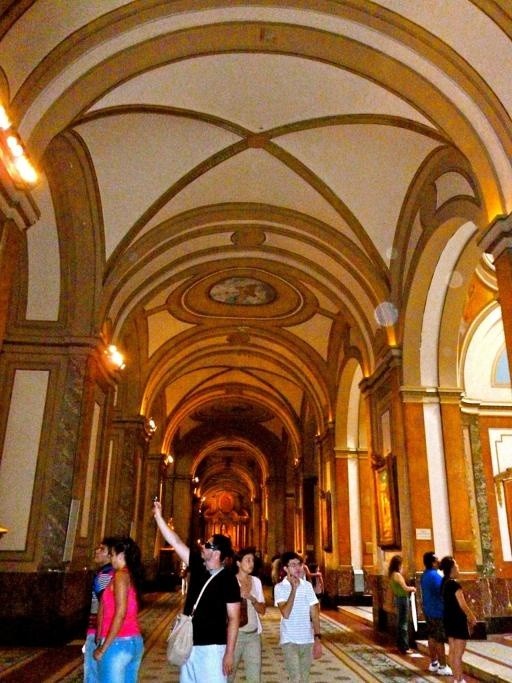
99;650;105;656
253;600;258;604
314;633;321;639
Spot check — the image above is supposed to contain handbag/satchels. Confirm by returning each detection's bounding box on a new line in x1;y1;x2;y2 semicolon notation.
239;597;248;627
167;613;193;666
180;568;187;578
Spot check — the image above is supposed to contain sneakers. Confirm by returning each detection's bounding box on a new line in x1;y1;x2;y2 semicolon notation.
429;662;453;676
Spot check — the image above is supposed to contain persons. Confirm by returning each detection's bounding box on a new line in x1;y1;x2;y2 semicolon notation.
422;552;455;676
274;551;322;682
83;538;115;682
96;537;144;683
388;556;419;653
299;557;322;580
306;562;325;610
179;558;191;596
153;497;241;682
232;547;266;681
270;554;283;601
437;556;476;683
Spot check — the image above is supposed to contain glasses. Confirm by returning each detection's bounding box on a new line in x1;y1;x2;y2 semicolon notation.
204;542;216;550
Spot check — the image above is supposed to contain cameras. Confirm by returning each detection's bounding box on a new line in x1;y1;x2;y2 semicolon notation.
152;495;158;504
